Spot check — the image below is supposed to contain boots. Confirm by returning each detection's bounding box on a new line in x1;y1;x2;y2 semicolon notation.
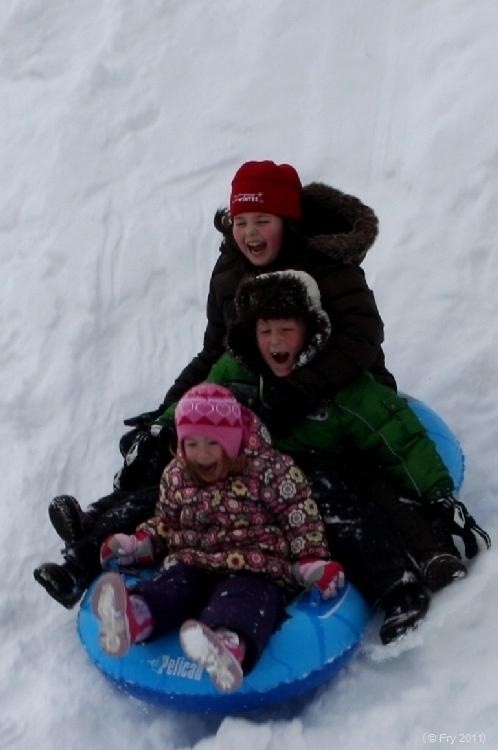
179;619;246;692
91;572;153;656
34;495;102;609
379;553;468;644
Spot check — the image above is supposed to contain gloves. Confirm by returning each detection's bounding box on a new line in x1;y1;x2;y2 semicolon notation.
293;555;344;601
100;531;153;568
431;495;491;560
119;404;167;458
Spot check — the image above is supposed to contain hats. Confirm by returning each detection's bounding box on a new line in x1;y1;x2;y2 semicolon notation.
230;160;302;219
227;269;331;370
175;383;242;463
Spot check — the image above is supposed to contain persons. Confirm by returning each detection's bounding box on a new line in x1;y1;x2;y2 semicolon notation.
33;269;429;647
48;160;492;592
91;384;346;692
